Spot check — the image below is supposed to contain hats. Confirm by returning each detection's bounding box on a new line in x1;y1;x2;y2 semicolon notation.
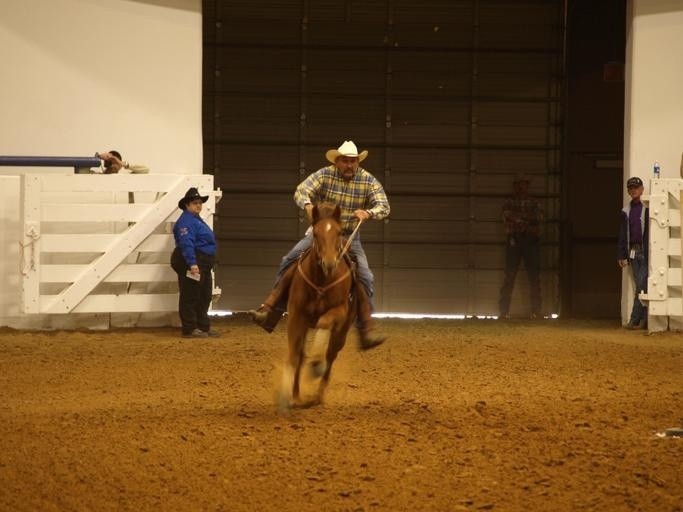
179;188;208;209
627;177;642;188
514;168;533;181
325;141;368;164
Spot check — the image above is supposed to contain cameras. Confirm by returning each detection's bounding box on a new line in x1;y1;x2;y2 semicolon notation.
101;160;112;172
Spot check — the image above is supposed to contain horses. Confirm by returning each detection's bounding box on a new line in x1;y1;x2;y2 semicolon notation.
279;203;358;408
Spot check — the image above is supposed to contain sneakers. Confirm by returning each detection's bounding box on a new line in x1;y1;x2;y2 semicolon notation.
249;310;278;334
623;323;640;330
208;330;220;337
360;333;386;349
183;329;207;337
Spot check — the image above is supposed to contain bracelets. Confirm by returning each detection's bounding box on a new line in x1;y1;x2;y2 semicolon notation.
124;160;130;170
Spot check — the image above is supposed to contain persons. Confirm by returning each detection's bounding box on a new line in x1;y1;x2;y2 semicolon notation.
165;187;226;340
72;151;151;226
617;173;650;329
497;170;548;319
250;141;393;350
